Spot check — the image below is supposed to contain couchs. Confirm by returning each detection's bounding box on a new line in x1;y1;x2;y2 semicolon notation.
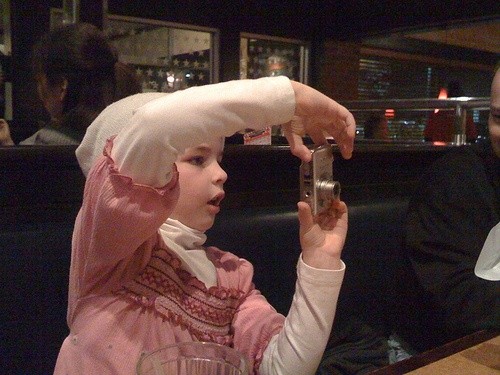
0;131;499;375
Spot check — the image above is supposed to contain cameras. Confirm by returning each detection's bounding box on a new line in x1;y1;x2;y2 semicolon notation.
299;144;341;217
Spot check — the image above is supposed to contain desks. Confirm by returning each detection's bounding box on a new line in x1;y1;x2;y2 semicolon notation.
363;315;499;375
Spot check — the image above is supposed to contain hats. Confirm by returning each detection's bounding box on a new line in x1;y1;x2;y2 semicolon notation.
75;92;169;180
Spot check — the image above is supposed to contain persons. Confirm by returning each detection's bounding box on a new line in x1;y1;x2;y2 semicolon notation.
53;76;356;375
0;22;143;146
399;67;500;354
